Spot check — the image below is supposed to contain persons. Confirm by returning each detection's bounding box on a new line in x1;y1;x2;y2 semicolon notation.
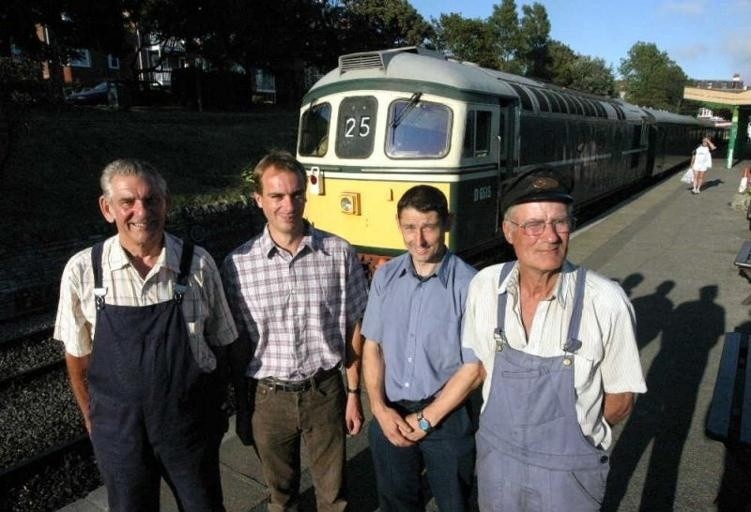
219;152;371;512
463;167;648;512
52;159;244;512
691;138;716;193
360;186;484;512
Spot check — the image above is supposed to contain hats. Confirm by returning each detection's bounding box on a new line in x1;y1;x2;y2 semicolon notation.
498;163;574;214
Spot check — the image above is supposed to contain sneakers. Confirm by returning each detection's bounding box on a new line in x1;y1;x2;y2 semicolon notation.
691;187;701;195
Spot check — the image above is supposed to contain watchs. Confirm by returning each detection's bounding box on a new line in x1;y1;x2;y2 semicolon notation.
417;411;435;433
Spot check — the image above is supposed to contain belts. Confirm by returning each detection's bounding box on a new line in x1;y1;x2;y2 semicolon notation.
263;371;338;393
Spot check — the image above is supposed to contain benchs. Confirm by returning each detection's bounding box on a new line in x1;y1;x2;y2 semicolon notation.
703;326;751;505
730;237;751;304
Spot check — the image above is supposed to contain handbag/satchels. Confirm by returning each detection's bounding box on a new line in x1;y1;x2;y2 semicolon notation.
680;168;694;184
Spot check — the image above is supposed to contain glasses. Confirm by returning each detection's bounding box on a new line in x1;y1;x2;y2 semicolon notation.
506;217;580;239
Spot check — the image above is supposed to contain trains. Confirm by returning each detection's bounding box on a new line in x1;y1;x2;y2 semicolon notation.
292;44;733;283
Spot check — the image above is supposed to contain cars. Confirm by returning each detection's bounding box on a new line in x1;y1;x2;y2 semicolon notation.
72;80;116;106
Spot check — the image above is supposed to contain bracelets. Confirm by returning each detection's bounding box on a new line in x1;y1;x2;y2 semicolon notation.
347;388;360;394
